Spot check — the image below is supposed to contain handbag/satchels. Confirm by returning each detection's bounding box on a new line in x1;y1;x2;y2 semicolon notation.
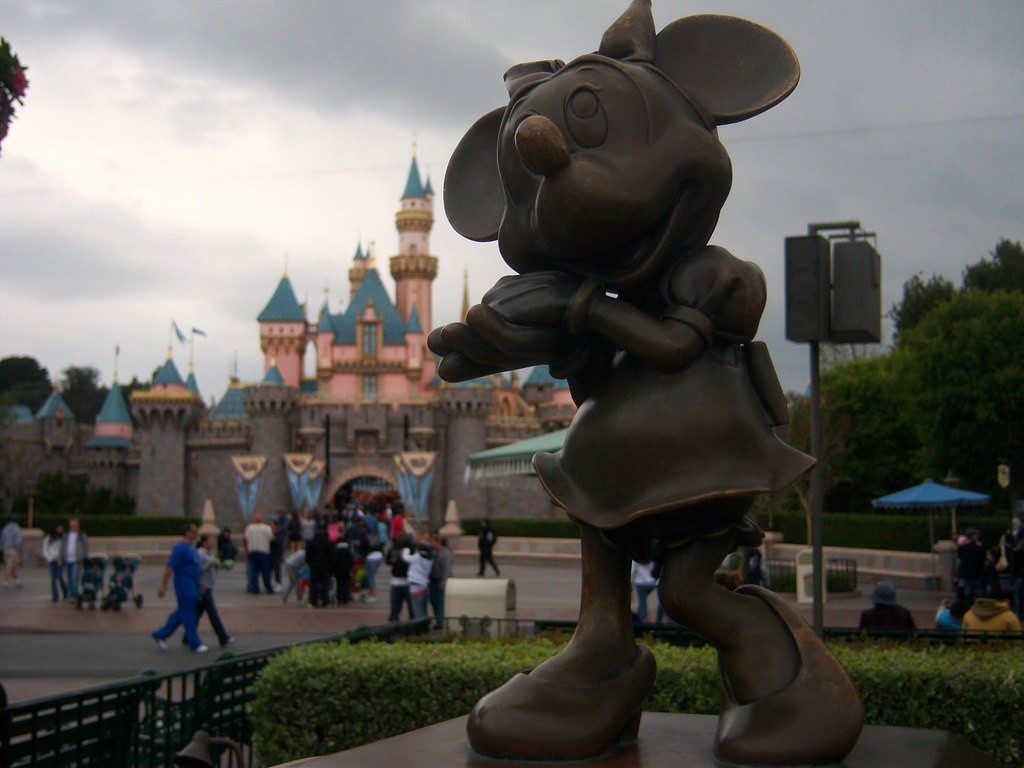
995;556;1008;571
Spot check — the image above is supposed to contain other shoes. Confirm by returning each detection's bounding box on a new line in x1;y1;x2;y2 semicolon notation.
195;645;208;653
434;626;443;630
223;636;237;648
156;638;167;649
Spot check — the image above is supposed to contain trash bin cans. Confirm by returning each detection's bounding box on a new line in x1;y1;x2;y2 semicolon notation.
443;577;517;641
796;548;825;604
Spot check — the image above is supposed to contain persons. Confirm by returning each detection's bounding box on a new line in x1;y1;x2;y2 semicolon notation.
385;530;452;630
217;528;240;563
151;523;209;653
183;534;235;647
723;547;771;587
116;566;131;587
857;581;917;629
41;524;67;602
478;518;500;576
629;561;660;622
60;518;89;601
90;564;102;576
656;606;675;625
935;516;1024;641
242;498;405;608
0;515;23;587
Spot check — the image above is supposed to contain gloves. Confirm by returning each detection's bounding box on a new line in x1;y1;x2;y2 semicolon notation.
210;557;220;568
224;560;234;569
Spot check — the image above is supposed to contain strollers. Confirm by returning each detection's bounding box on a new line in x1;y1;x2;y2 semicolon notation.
76;554;108;609
102;553;143;613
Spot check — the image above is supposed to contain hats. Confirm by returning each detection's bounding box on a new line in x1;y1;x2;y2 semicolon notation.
966;527;978;536
870;582;896;604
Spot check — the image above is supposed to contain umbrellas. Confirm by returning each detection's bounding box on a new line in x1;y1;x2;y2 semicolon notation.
465;427;568;482
871;479;990;543
